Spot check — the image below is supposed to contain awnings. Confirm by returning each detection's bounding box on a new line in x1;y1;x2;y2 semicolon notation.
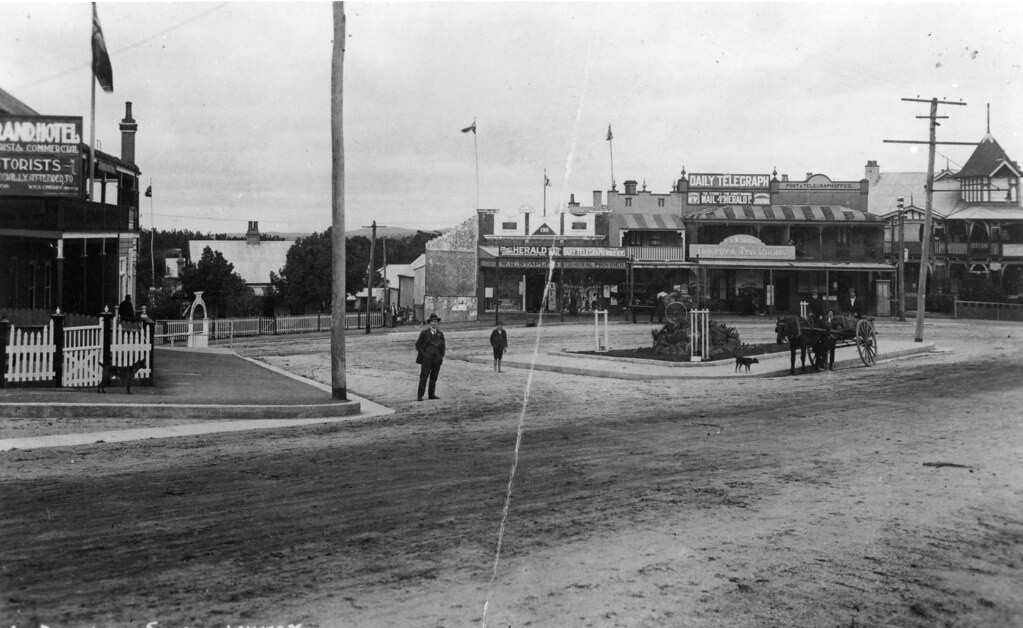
698;254;898;273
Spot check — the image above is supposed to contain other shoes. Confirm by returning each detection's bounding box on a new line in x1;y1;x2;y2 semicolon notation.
429;395;440;399
418;396;423;401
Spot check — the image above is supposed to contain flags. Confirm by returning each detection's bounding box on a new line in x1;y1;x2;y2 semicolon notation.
145;185;152;197
92;12;113;92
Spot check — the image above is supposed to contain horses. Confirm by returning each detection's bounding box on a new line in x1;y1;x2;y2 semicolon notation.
776;316;830;375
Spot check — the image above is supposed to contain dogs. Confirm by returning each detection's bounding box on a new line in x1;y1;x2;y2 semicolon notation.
97;359;147;394
735;355;759;373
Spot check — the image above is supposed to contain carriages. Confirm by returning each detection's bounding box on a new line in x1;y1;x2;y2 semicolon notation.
773;311;878;377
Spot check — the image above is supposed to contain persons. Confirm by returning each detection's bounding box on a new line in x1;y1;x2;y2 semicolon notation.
118;294;136;321
846;288;861;319
823;309;842;370
490;321;508;373
414;312;446;401
647;297;655;322
657;297;663;322
807;289;824;343
390;302;398;328
139;306;154;325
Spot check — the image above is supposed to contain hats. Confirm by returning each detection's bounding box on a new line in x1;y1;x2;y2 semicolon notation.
848;288;856;294
826;308;836;315
124;294;132;299
427;313;441;324
